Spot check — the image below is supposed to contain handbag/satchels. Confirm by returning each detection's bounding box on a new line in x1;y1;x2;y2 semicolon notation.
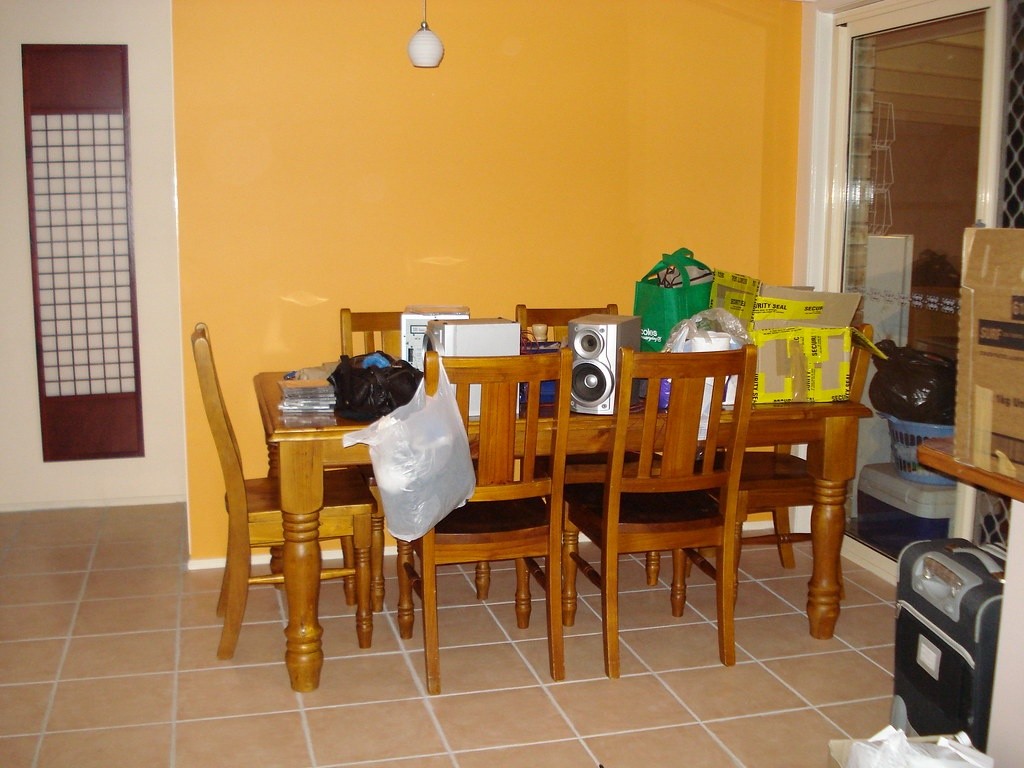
327;351;424;423
633;247;714;353
520;329;563;406
344;354;477;542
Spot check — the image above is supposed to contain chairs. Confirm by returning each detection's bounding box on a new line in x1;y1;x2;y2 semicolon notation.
397;349;572;693
339;307;402;359
666;323;874;617
579;346;754;679
191;322;374;660
516;304;618;343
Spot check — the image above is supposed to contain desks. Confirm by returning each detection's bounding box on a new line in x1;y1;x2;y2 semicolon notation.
915;431;1024;503
254;370;856;691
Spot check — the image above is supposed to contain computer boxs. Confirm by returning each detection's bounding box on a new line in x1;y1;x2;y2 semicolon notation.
401;304;470;372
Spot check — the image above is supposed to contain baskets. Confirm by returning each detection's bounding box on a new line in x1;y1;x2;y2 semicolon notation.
871;410;956;487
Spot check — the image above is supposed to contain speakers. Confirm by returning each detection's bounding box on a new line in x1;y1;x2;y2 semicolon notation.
423;318;520;422
568;314;641;415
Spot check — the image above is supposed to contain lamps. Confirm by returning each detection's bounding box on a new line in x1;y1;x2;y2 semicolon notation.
407;5;446;69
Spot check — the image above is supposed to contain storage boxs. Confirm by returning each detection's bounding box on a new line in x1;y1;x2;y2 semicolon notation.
955;228;1024;481
707;269;862;404
860;461;1001;557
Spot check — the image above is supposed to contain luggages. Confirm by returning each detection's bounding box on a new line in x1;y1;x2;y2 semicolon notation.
892;537;1006;754
